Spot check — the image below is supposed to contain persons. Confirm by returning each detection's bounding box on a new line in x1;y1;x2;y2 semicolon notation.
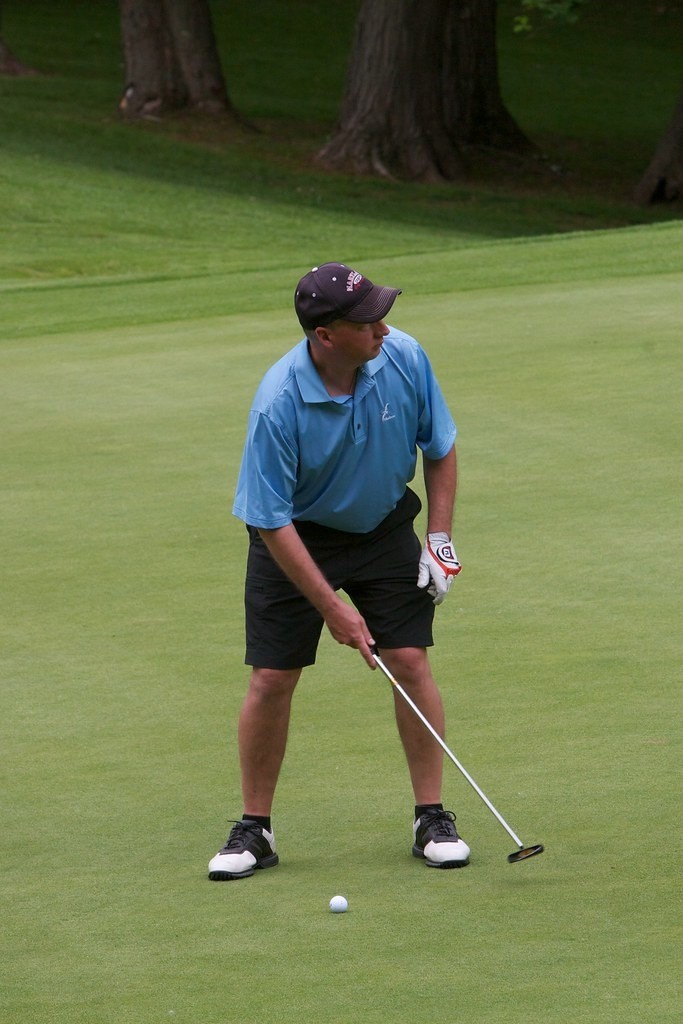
208;260;472;882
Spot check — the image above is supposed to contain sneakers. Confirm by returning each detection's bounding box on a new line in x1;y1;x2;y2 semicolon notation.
208;820;279;880
413;805;470;869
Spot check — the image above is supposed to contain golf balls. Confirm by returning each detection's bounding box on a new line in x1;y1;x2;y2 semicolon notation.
328;895;348;913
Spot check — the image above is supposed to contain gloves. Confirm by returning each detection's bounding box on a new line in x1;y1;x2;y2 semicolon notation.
417;531;462;605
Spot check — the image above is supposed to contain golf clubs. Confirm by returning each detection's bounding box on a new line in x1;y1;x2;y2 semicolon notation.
368;644;544;864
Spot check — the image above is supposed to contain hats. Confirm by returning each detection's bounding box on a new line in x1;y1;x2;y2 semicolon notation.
294;262;403;330
